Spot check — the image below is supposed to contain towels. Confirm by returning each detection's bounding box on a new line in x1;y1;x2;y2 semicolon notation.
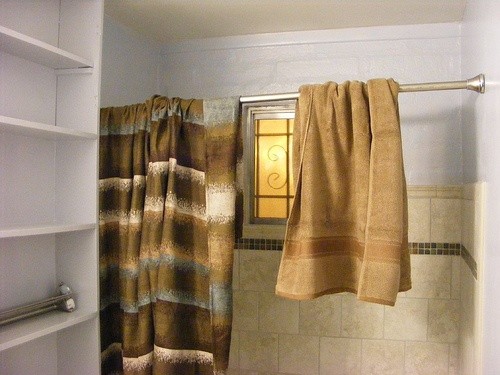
275;78;413;306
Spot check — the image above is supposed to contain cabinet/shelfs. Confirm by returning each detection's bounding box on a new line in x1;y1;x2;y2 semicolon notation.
0;0;101;374
